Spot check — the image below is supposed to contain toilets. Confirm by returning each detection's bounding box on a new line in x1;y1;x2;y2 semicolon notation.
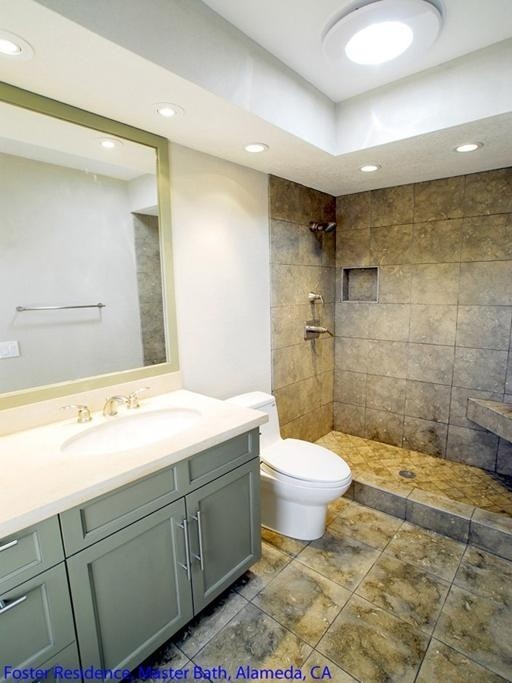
226;392;351;541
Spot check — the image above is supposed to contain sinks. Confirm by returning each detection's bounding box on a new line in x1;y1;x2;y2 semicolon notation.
60;408;202;455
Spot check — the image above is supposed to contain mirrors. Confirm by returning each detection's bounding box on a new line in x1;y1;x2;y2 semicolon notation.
1;81;185;411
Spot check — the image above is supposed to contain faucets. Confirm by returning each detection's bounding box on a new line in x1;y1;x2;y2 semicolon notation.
102;395;129;417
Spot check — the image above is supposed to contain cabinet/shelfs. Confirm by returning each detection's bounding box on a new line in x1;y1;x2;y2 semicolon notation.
56;423;261;683
0;515;84;683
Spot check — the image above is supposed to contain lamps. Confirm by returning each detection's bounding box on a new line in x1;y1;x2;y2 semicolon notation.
321;2;443;78
